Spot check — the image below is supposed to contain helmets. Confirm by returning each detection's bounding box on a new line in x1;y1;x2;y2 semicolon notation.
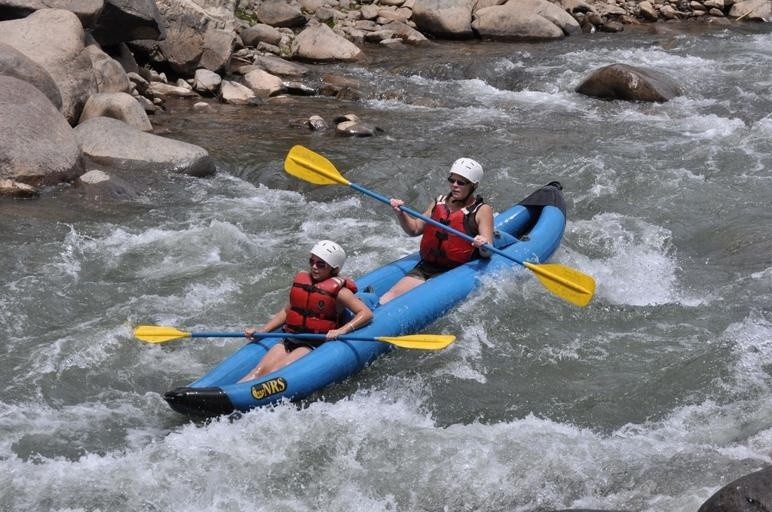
310;239;346;274
449;157;483;188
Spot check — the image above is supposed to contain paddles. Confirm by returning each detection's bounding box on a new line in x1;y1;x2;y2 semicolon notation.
284;145;595;308
134;324;455;351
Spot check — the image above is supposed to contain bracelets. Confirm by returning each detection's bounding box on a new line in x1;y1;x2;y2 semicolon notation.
346;321;356;330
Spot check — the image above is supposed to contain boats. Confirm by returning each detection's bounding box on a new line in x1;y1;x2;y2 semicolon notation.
163;182;565;420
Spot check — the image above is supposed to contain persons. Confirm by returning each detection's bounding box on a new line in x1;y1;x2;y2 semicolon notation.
361;157;497;307
237;237;375;383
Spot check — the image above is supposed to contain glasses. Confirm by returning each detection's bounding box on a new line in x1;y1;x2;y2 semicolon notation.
448;176;471;186
309;258;326;269
